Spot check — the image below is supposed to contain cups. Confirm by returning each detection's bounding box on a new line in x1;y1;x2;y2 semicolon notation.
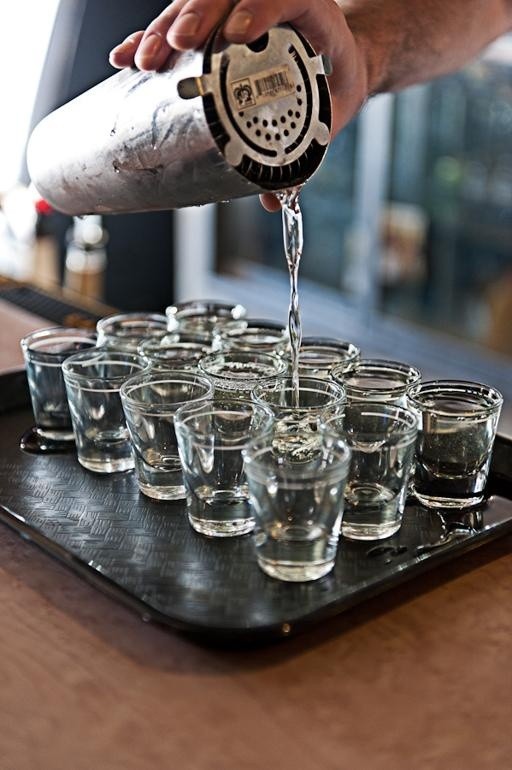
403;378;504;510
19;293;421;583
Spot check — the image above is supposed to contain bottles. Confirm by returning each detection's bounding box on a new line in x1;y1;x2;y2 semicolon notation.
25;14;335;221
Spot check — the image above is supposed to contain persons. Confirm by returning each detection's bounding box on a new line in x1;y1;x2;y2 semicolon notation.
107;0;512;215
